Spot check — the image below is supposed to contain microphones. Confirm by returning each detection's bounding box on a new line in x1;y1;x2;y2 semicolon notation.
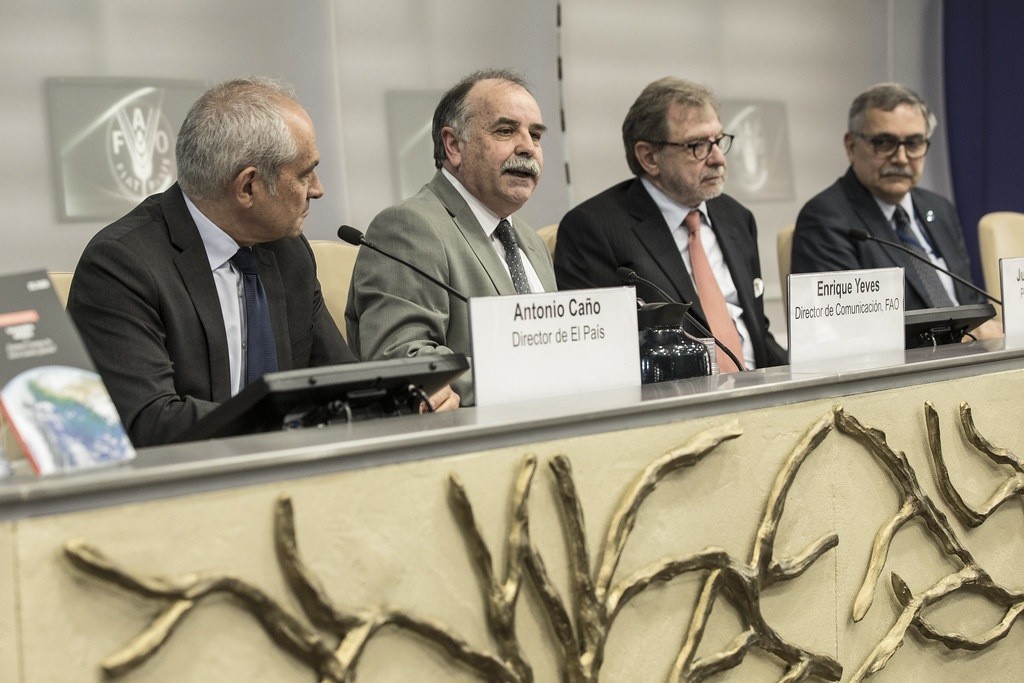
337;225;468;303
616;267;746;374
848;228;1002;305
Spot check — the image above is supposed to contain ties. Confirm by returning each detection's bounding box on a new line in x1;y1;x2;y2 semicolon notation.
230;247;278;392
892;203;956;308
493;219;533;295
684;211;747;374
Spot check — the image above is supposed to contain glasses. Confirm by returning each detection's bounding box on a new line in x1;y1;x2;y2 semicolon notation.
848;131;931;160
633;133;735;161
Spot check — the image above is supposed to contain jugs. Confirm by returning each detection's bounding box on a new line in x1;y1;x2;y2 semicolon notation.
637;297;711;384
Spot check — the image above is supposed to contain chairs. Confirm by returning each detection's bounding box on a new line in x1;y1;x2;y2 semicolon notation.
977;210;1024;325
306;239;362;346
777;225;796;324
536;223;559;255
46;272;75;311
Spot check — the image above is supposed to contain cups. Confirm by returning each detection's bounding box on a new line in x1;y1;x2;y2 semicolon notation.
697;338;720;375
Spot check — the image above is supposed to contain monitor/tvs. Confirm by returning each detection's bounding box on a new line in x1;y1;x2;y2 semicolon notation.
905;305;997;349
169;353;471;444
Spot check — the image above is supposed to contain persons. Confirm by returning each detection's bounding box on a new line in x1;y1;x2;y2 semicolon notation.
343;69;558;408
554;76;789;378
65;79;462;450
790;82;1004;349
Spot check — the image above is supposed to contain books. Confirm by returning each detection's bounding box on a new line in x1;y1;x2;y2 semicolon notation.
0;270;137;477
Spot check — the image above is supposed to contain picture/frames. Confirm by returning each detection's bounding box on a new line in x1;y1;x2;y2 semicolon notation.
717;96;798;205
47;76;203;223
387;90;446;203
